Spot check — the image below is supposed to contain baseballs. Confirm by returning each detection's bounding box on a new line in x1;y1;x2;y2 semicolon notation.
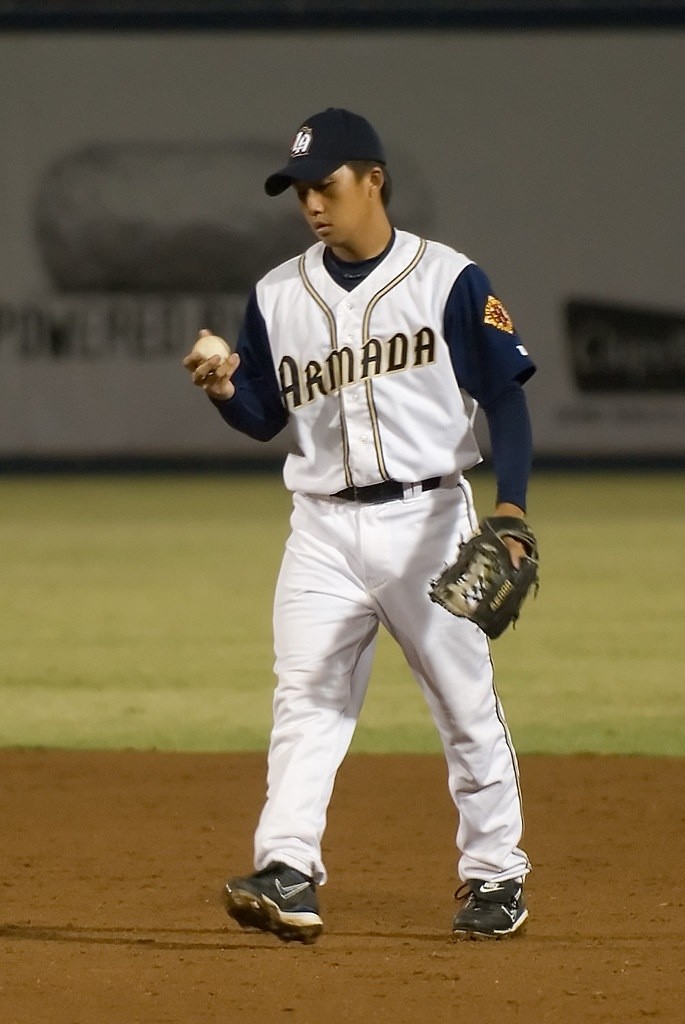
193;335;231;372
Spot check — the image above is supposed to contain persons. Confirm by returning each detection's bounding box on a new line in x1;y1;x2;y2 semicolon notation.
182;106;540;943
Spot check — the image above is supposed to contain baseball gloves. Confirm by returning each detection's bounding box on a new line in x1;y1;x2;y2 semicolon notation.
428;515;540;640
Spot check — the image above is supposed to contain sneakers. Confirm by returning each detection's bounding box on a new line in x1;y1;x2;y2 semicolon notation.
223;860;325;946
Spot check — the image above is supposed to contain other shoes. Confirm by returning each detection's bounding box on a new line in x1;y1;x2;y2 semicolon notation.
452;877;529;941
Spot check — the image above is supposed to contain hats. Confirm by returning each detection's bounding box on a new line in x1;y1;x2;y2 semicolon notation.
264;108;387;197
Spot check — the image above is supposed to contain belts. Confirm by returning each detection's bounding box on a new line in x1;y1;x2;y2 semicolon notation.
329;477;441;505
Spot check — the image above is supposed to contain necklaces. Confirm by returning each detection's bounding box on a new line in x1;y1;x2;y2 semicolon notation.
326;229;396;279
